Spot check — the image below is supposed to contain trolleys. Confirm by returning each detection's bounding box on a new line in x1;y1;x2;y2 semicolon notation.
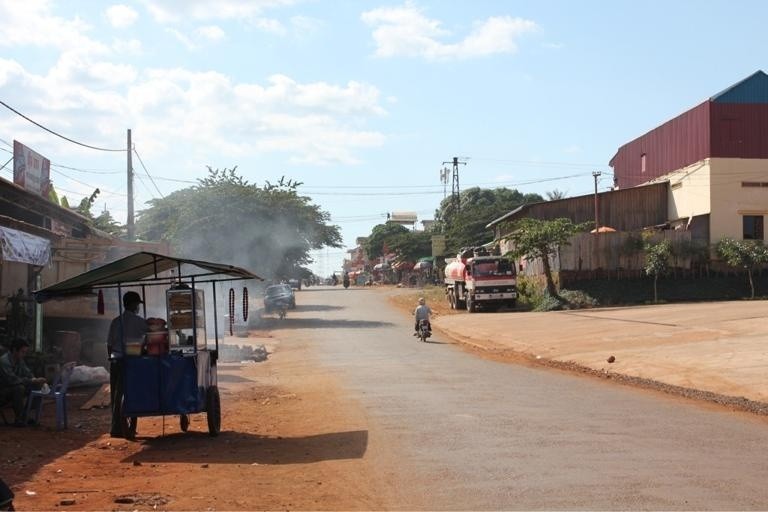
28;250;267;441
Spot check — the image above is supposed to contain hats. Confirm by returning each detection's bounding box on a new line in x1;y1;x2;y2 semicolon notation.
123;291;144;303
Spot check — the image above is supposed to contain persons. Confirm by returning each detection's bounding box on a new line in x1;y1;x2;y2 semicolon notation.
342;271;349;288
412;298;435;337
105;290;152;439
1;336;50;428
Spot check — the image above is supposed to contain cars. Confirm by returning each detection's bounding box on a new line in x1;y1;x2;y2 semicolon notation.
262;283;296;312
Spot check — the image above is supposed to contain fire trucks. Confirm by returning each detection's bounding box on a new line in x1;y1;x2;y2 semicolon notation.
444;246;519;314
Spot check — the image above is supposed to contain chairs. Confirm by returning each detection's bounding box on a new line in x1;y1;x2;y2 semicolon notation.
23;358;78;432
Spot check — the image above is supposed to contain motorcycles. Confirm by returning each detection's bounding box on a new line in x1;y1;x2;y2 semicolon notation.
343;280;350;289
412;312;433;343
